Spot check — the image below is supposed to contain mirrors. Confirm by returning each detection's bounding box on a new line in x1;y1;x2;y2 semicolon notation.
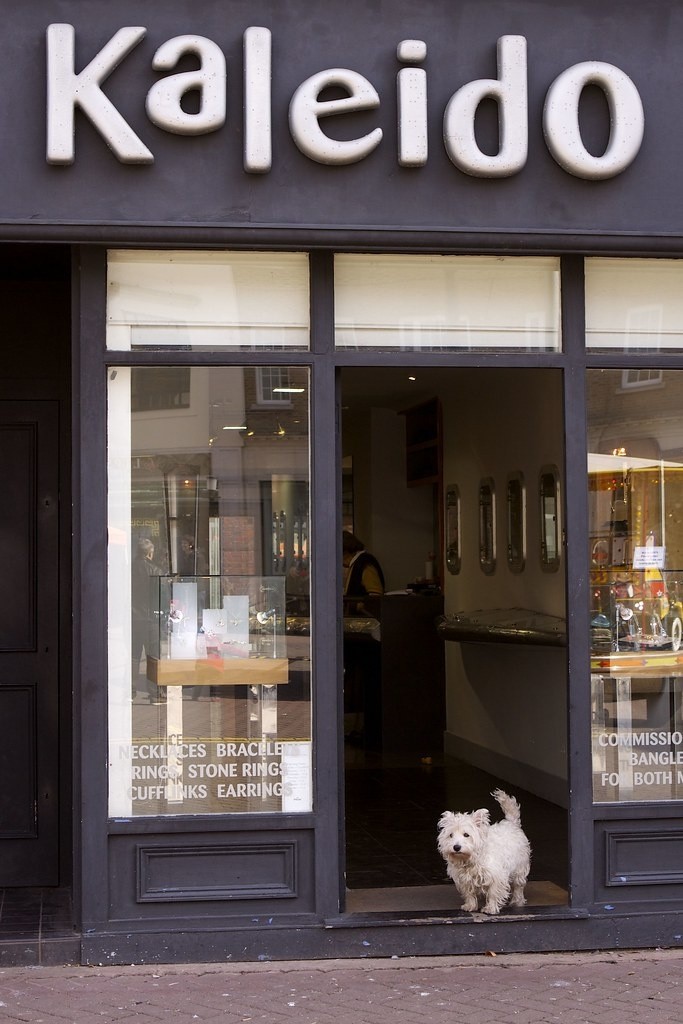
536;464;559;573
477;477;497;575
444;484;461;576
506;469;525;574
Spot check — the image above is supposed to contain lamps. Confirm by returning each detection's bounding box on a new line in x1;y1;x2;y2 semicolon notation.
257;608;278;658
165;608;182;658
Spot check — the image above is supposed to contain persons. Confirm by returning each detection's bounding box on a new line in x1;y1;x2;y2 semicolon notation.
343;529;383;748
132;535;222;704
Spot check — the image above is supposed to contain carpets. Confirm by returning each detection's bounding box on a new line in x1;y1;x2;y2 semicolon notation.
344;879;570;911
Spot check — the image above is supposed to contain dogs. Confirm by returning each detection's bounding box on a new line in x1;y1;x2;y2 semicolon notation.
437;789;531;915
